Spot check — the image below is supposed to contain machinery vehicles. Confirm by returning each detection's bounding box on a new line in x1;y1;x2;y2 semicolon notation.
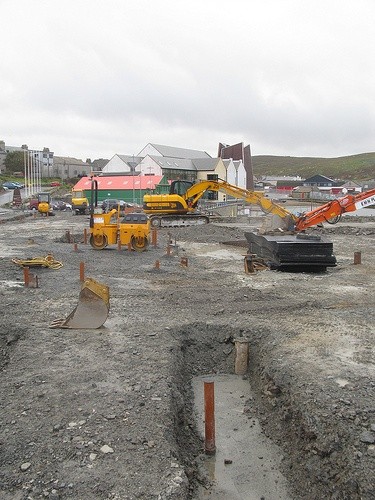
72;188;90;215
295;188;375;230
142;177;297;229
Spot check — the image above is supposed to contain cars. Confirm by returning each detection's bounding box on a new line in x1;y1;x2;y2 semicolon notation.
93;199;133;212
3;181;24;189
50;181;62;187
